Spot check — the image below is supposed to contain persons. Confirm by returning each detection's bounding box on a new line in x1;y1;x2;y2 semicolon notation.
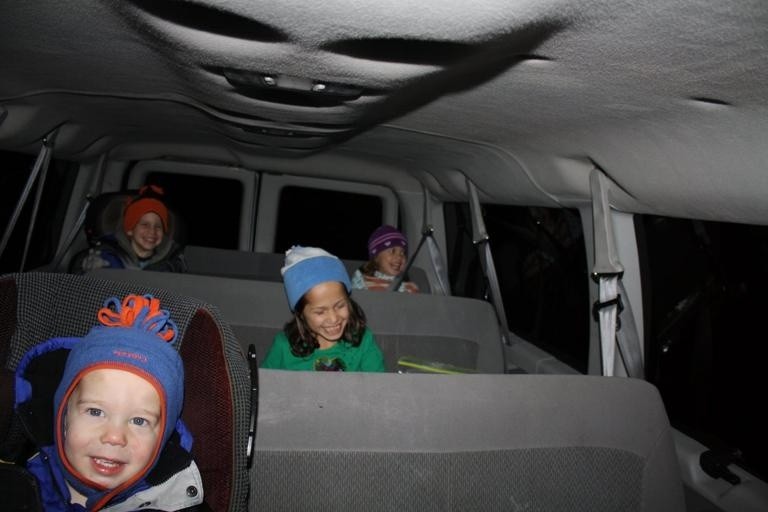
1;293;213;511
348;227;423;293
79;189;189;273
257;254;386;372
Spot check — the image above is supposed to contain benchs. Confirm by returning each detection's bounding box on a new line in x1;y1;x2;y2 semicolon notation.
1;239;686;510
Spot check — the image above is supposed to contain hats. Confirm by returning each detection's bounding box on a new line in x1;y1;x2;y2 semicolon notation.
123;186;168;233
368;225;408;257
53;326;185;490
281;246;351;312
